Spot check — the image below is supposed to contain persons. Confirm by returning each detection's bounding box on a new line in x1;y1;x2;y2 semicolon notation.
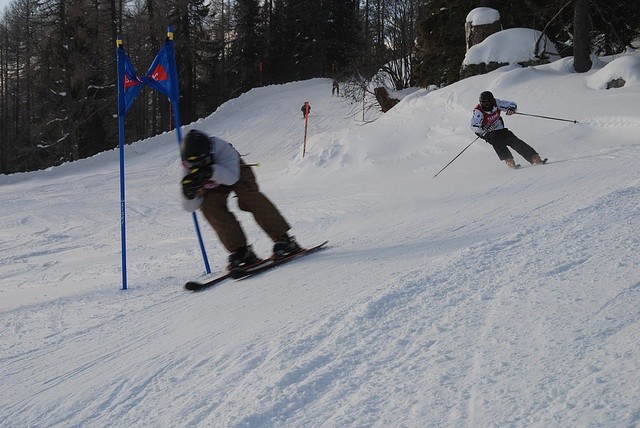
180;130;301;270
332;78;339;95
471;91;548;169
301;102;310;119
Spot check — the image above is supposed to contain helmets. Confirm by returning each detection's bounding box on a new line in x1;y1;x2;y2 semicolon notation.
479;91;497;111
181;130;210;163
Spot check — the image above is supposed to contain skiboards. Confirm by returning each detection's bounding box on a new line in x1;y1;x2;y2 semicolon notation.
184;240;329;291
515;157;548;169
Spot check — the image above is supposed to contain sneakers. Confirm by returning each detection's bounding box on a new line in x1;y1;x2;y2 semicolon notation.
226;245;258;270
532;155;542;164
506;159;515;167
272;236;299;260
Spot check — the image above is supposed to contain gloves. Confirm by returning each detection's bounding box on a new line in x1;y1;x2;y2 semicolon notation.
506;106;516;115
484;125;494;133
182;166;213;199
